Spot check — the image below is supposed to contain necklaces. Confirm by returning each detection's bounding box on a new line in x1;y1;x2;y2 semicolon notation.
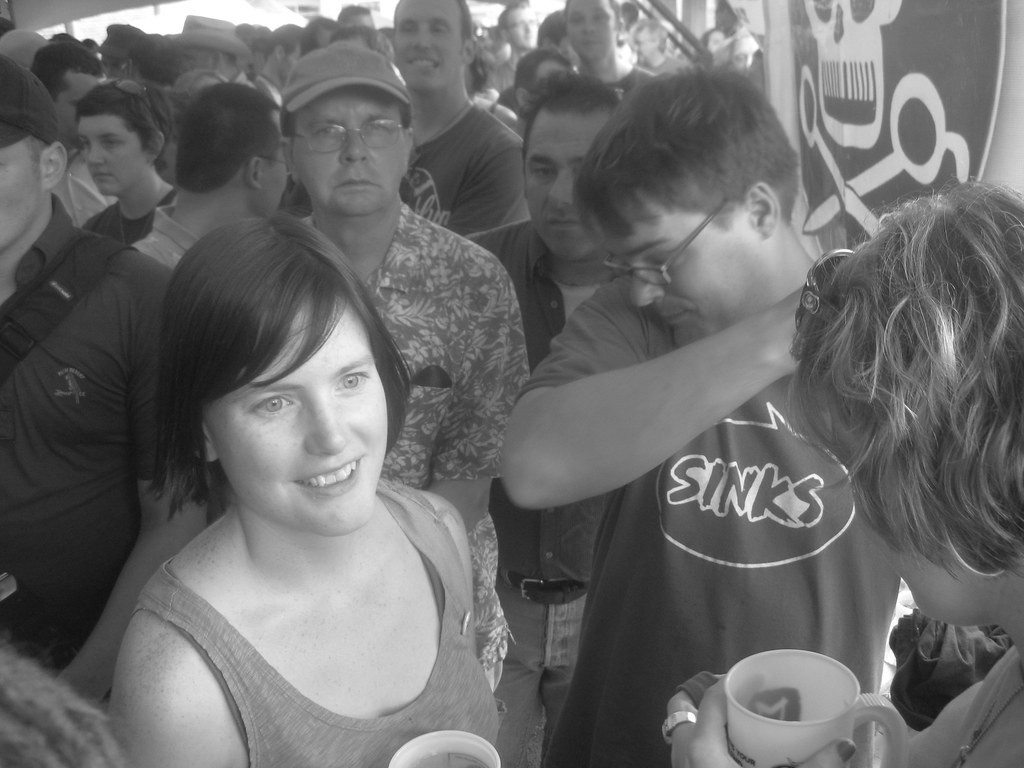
409;97;470;164
959;686;1024;762
119;181;164;243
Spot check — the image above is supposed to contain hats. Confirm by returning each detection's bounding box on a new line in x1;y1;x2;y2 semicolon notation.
0;54;59;146
0;29;49;70
178;15;255;65
280;42;410;111
98;24;145;60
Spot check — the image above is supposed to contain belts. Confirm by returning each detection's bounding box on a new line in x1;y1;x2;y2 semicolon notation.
495;567;589;605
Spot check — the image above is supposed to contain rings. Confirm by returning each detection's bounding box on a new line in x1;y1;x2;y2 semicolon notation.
662;712;696;745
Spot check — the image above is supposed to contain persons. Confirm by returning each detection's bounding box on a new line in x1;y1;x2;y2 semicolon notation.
0;0;1024;768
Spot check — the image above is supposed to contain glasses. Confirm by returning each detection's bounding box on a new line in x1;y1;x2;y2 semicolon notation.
97;76;160;128
794;249;856;336
602;197;730;285
289;119;404;154
258;155;296;174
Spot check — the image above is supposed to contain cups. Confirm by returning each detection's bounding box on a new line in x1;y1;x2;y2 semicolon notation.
725;649;908;768
388;730;501;768
